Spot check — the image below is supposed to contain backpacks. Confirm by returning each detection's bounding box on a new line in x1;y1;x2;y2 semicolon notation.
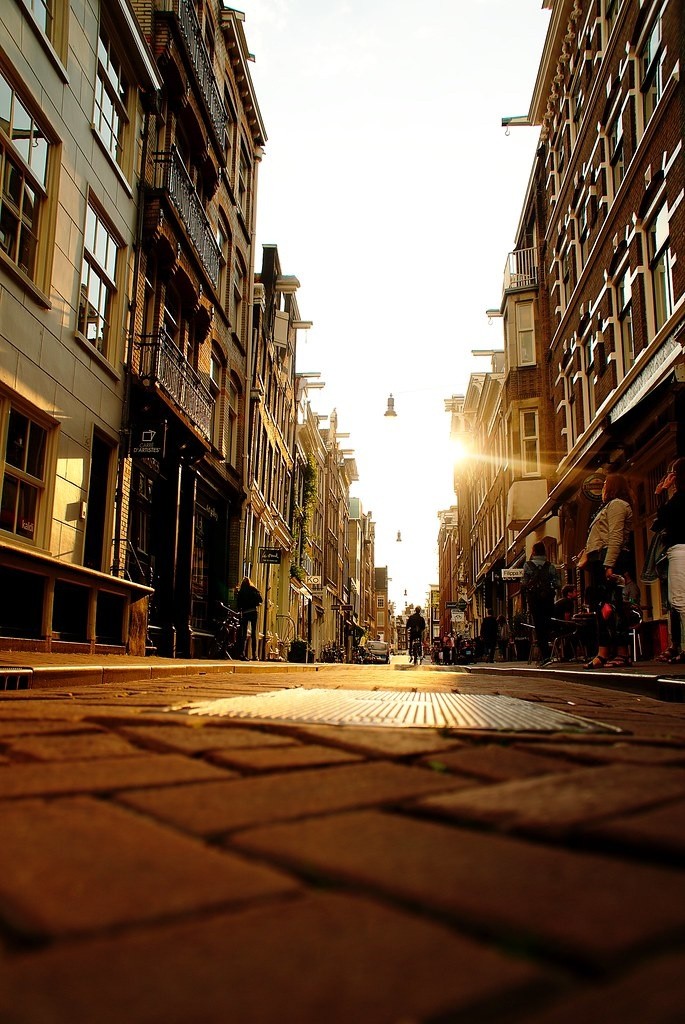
525;560;556;601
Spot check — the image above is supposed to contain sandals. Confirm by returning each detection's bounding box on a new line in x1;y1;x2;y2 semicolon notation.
655;647;685;664
583;654;632;669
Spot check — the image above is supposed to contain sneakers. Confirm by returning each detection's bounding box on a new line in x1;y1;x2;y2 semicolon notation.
536;658;552;668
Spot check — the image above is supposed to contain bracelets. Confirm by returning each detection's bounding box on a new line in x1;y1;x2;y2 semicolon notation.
605;566;612;570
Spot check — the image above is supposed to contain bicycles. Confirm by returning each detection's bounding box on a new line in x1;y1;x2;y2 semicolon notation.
208;603;242;659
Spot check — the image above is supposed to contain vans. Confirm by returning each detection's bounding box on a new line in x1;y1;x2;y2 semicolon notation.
367;641;390;663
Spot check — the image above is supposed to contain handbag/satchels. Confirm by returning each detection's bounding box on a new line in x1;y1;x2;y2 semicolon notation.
576;547;593;572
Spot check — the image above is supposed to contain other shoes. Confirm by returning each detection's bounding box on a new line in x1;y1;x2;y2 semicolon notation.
241;656;250;661
251;656;260;661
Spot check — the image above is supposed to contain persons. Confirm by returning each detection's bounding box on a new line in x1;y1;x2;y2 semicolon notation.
433;633;458;666
406;606;426;666
522;541;561;667
641;458;685;664
480;610;498;663
235;575;262;662
496;614;511;663
574;472;634;670
